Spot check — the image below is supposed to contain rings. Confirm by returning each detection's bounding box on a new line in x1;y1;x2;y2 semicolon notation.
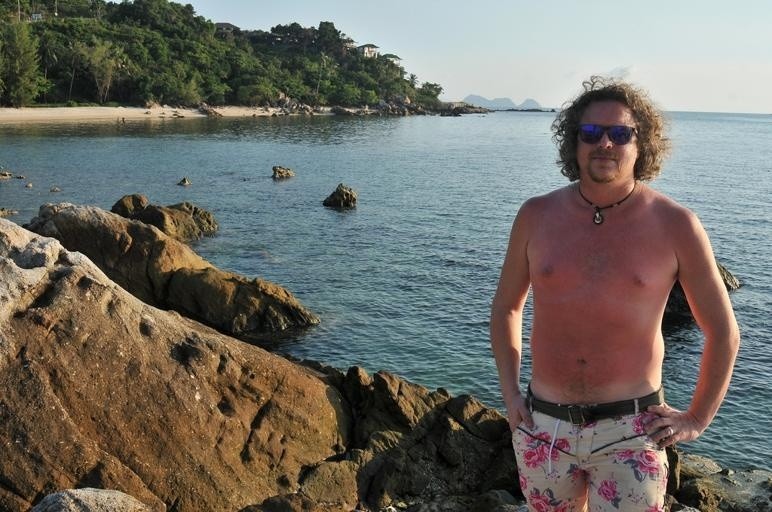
667;426;674;436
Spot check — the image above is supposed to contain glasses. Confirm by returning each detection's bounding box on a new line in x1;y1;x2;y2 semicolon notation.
575;124;640;146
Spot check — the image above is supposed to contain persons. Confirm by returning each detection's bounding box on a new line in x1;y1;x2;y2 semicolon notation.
489;74;740;512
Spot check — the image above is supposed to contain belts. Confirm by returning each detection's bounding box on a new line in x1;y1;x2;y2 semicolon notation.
525;381;663;425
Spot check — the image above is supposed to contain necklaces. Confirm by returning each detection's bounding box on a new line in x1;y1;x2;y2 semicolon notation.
578;182;636;225
